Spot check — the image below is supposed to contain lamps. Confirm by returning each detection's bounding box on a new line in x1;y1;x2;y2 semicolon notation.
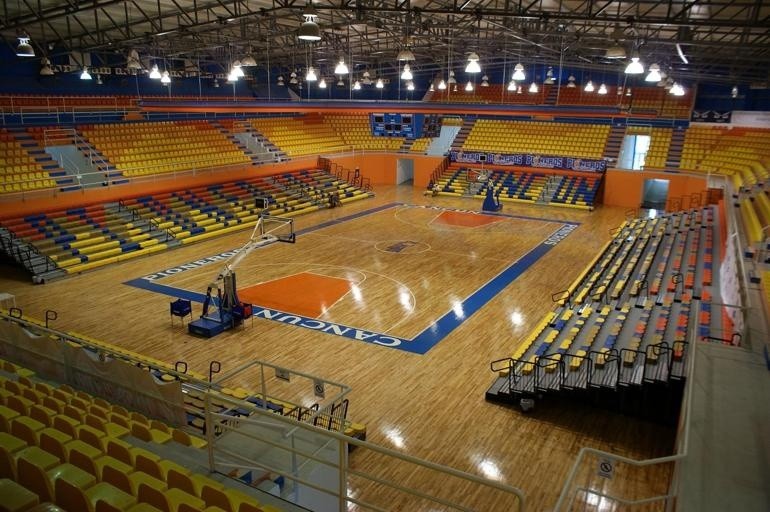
393;12;417;64
297;1;323;43
602;19;627;61
10;13;56;77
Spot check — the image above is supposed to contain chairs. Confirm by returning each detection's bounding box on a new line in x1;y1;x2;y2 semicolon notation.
0;114;252;191
644;119;770;176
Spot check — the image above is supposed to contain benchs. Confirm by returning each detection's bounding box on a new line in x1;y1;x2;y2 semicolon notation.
498;206;715;374
427;166;603;213
